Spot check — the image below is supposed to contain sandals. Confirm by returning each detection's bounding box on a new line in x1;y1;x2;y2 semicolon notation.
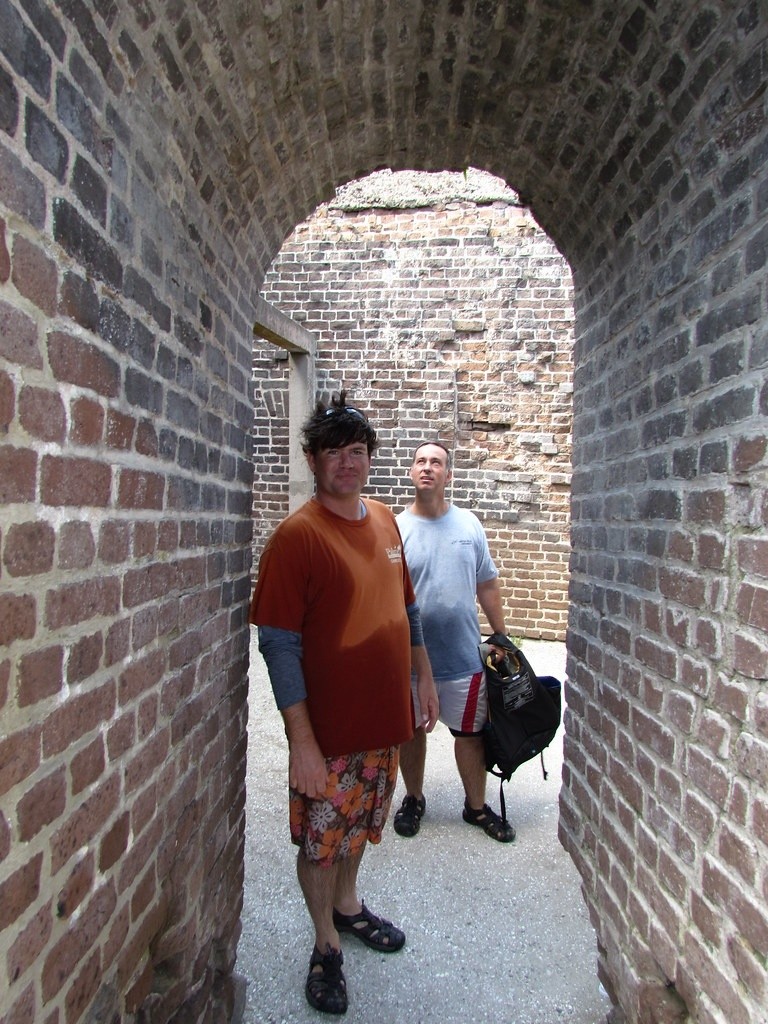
333;898;405;953
305;942;348;1013
394;794;426;836
463;797;516;842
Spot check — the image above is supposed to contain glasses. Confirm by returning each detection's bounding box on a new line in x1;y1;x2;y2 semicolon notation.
318;407;366;422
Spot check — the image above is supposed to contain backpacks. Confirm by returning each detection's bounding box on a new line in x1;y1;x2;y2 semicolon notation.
484;631;560;779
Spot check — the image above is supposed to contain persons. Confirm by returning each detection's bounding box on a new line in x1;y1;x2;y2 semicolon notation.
249;388;441;1015
394;442;517;842
